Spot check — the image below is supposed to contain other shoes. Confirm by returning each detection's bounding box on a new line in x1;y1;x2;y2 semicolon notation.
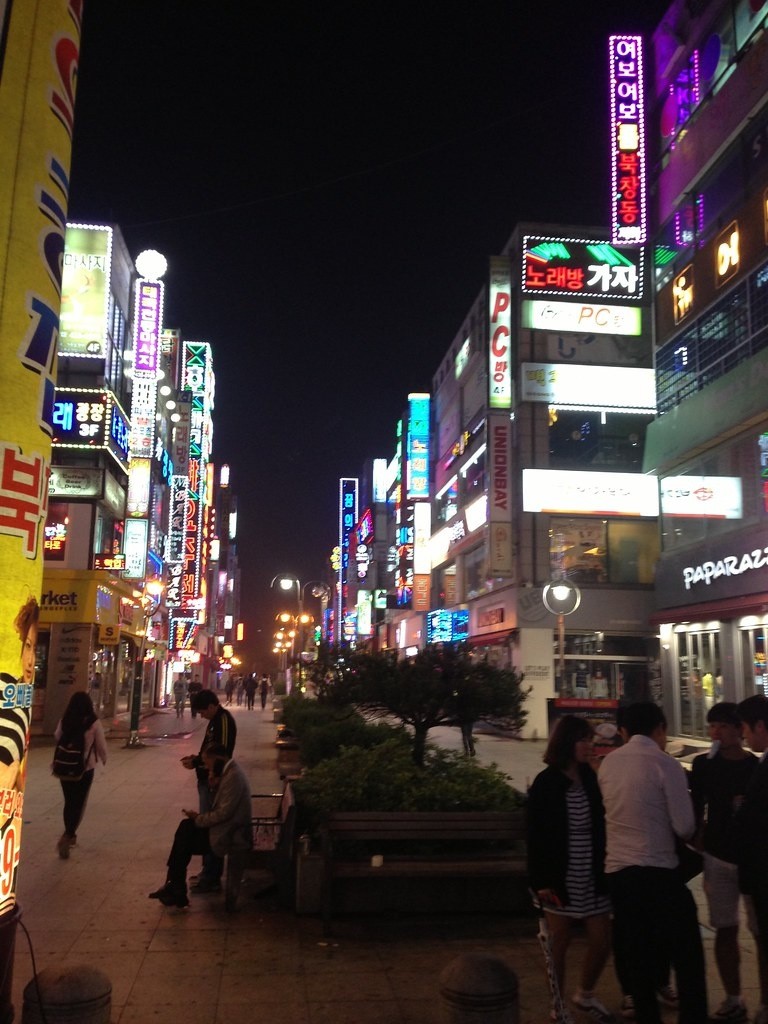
58;840;71;859
190;880;222;893
69;835;77;847
189;875;199;881
149;887;188;908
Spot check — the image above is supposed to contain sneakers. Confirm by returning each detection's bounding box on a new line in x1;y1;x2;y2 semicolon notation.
551;1009;576;1024
571;993;617;1024
654;987;678;1008
714;1001;747;1020
618;993;634;1017
754;1005;768;1024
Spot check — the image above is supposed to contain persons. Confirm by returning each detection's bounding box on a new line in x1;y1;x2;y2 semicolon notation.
178;690;238;894
173;672;188;719
54;691;108;860
188;674;203;718
688;696;768;1024
596;701;711;1024
524;713;617;1020
146;744;252;900
225;673;272;711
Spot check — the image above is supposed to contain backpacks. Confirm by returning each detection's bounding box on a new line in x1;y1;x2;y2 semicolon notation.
51;713;98;778
261;679;268;695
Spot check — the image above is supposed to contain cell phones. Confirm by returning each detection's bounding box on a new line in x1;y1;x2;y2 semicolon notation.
213;759;225;777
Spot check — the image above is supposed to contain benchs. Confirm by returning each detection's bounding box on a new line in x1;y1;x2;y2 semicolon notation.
225;779;297;912
322;808;528;939
